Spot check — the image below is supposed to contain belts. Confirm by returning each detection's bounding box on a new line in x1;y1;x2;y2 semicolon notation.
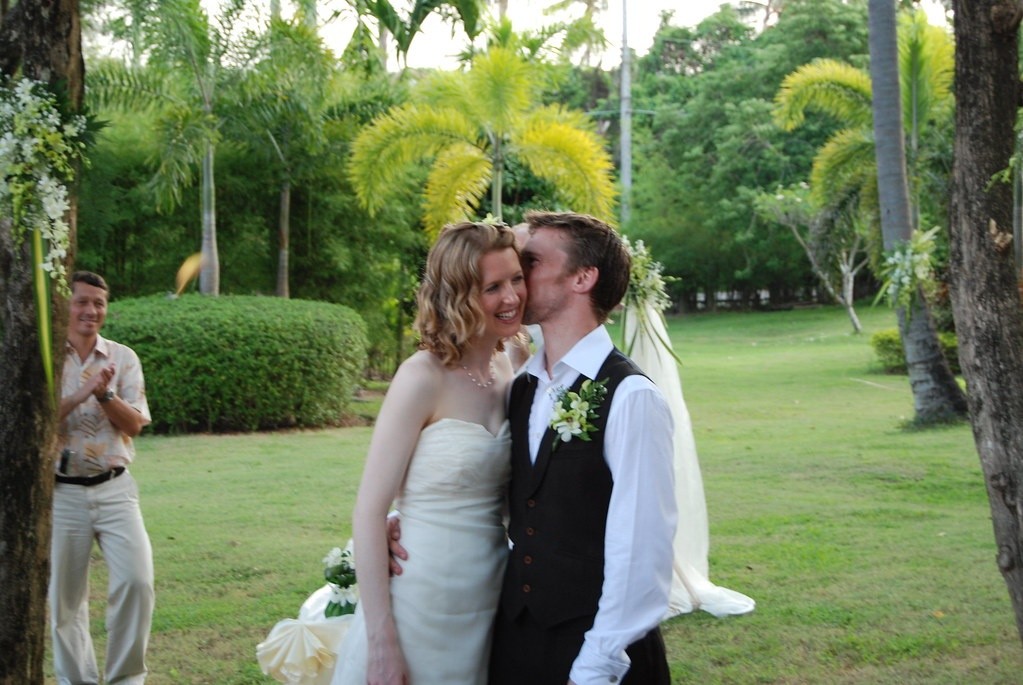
55;466;125;486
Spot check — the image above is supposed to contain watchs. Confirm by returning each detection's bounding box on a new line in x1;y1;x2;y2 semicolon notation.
98;389;115;402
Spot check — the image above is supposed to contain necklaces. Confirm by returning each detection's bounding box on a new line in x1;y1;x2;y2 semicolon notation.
459;357;499;387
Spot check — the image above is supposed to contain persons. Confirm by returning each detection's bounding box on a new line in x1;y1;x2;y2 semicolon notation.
387;210;681;685
256;216;536;685
49;270;153;685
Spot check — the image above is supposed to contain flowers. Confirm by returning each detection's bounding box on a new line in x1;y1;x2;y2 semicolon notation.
475;212;504;227
869;223;942;336
623;233;685;371
325;538;357;618
0;65;113;416
547;379;609;450
